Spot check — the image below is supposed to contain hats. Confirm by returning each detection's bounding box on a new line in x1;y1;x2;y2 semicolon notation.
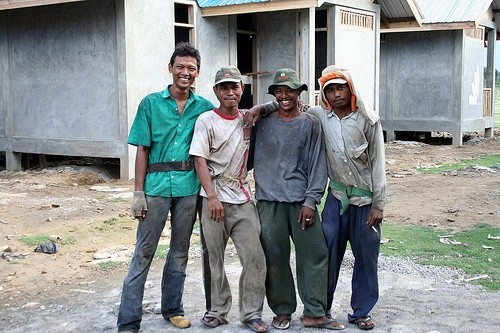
266;68;309;95
213;65;242;87
320;65;351;91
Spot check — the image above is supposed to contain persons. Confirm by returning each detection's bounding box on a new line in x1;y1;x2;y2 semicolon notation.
188;66;310;332
117;41;217;333
244;65;388;329
246;69;345;330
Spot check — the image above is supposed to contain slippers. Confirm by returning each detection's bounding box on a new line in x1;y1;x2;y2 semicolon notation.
302;316;346;330
272;314;291;329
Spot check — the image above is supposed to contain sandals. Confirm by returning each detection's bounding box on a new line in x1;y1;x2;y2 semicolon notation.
244;318;269;333
353;316;375;330
201;316;220;328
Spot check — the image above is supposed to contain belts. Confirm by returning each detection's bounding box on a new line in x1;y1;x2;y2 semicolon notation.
145;161;195;174
329;180;373;216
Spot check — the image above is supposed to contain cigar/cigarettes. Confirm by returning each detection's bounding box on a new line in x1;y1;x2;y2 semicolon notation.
372;226;377;232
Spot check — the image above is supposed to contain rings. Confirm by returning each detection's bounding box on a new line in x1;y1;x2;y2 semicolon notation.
306;219;310;221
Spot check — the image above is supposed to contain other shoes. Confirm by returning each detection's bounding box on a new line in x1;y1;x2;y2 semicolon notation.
167;315;191;328
